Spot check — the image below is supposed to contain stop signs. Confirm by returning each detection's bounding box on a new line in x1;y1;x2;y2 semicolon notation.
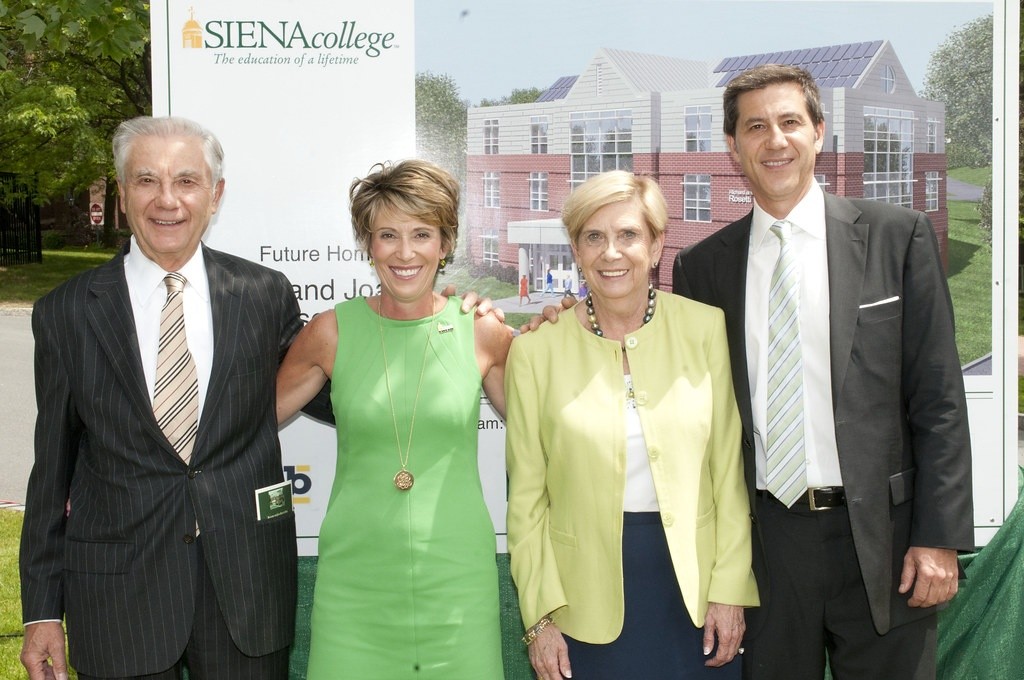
90;204;103;224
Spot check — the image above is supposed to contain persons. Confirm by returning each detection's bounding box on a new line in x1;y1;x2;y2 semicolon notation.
540;270;554;297
564;276;572;297
520;64;974;680
579;283;587;298
277;160;514;679
520;275;531;304
505;172;760;680
16;118;504;680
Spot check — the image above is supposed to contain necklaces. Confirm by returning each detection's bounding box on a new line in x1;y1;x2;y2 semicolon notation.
378;291;435;486
586;283;657;350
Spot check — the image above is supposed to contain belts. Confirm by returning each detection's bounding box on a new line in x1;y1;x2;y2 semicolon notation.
755;487;848;511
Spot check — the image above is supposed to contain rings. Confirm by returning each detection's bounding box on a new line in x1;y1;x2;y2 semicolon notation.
736;647;744;654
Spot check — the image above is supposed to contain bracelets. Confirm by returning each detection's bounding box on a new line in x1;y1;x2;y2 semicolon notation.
522;616;553;645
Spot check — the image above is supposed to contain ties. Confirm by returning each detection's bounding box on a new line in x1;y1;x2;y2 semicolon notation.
152;271;200;538
766;219;808;509
